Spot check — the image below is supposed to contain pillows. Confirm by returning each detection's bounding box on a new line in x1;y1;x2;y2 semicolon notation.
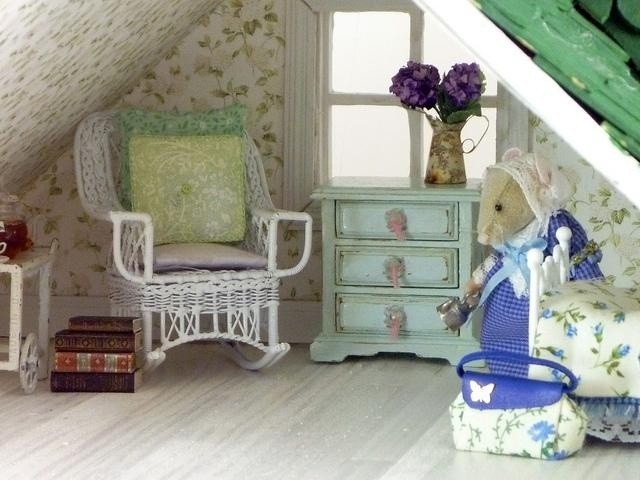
115;102;250;212
128;134;246;245
133;242;268;274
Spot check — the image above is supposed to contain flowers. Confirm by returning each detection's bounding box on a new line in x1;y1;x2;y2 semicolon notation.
388;60;487;124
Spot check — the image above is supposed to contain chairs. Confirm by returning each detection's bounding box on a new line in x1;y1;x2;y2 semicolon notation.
531;205;640;444
73;108;313;377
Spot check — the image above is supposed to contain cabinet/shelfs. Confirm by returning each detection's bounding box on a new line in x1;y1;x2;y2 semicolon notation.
309;175;486;368
0;237;60;395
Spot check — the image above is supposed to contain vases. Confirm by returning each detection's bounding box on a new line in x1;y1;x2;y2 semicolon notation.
423;115;489;184
